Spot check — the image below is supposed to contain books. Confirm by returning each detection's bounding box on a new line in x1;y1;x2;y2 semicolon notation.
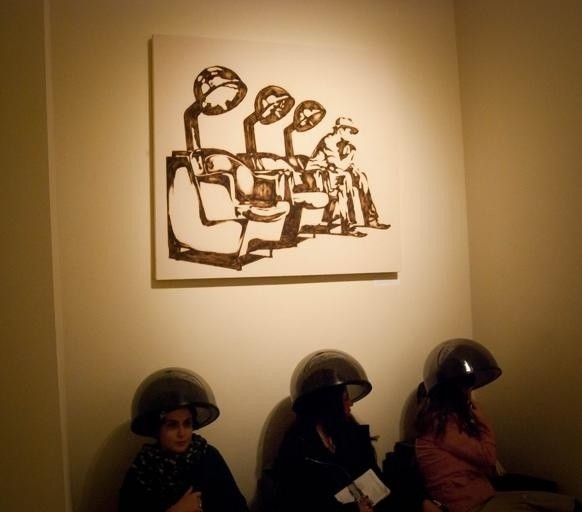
333;468;391;510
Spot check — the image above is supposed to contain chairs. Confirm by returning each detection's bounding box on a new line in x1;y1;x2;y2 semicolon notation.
164;147;355;272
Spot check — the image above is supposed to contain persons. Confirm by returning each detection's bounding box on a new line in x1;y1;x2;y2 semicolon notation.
255;367;395;512
304;115;391;238
116;390;249;511
412;358;582;512
